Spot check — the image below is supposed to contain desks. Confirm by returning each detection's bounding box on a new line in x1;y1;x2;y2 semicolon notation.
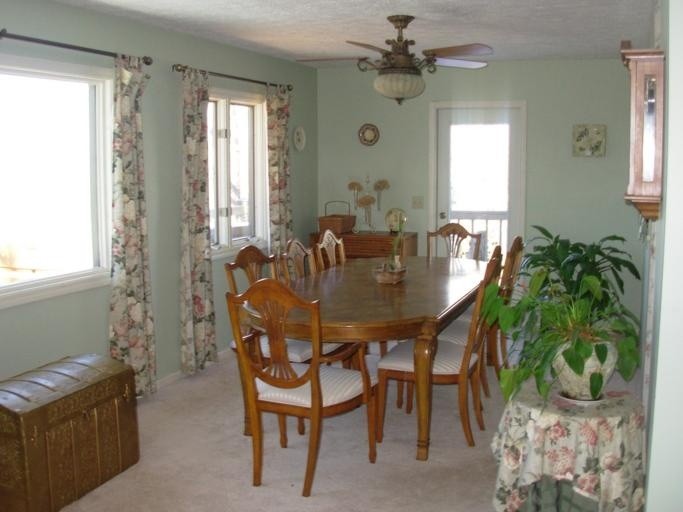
488;370;648;510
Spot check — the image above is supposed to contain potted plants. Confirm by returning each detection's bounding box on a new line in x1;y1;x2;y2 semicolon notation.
477;263;642;400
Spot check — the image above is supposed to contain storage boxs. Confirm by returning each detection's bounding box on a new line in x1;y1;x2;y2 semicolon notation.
0;353;140;511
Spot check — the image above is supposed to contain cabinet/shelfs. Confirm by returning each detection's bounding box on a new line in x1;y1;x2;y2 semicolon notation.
621;49;664;221
312;231;417;269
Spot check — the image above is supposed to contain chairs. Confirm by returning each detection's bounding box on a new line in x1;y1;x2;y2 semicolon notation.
374;245;504;464
456;235;526;388
226;280;378;495
224;246;356;451
274;239;369;375
315;229;346;272
426;223;482;258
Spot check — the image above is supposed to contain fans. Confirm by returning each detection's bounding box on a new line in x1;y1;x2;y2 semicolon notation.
296;15;495;105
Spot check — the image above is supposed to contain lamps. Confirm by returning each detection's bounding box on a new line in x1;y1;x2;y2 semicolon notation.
374;67;427;105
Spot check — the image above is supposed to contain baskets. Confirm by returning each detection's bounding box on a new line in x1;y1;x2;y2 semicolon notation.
318;200;356;234
